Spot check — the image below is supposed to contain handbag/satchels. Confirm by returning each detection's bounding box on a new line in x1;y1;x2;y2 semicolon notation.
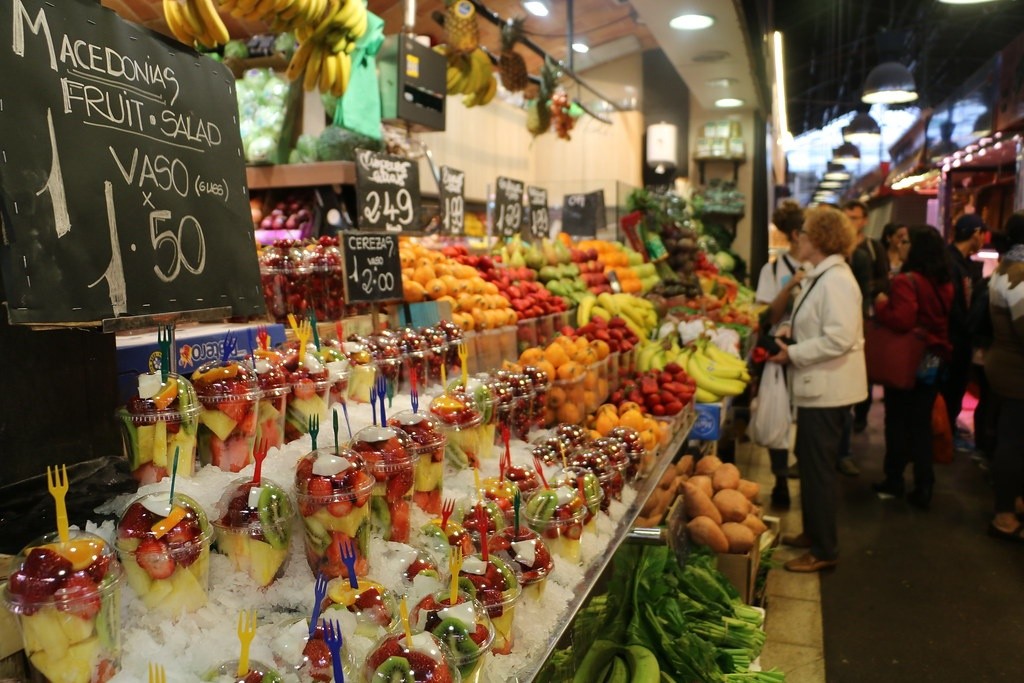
863;315;926;391
745;361;793;452
931;389;953;463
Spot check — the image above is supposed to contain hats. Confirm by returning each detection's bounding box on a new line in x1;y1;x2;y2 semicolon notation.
955;215;986;242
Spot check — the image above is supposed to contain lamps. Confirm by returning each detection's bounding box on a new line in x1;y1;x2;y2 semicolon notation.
834;135;862;163
806;161;850;206
930;103;959;162
841;104;881;136
571;35;591;53
860;61;919;104
645;120;679;175
885;147;941;190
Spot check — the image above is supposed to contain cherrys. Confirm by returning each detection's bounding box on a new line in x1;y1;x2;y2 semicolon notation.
348;322;645;515
257;235;351;328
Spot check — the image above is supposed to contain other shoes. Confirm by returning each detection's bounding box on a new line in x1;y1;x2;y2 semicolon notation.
788;464;801;478
771;486;788;508
872;476;931;509
853;417;866;431
987;493;1024;544
971;448;993;470
953;426;971;448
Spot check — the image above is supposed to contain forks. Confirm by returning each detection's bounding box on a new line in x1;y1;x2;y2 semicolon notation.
17;345;582;683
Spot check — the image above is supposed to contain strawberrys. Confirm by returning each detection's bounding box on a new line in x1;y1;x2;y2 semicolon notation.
558;314;697;414
441;243;568;349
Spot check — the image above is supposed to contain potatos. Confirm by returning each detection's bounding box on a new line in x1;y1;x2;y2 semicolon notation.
635;454;695;530
678;455;768;550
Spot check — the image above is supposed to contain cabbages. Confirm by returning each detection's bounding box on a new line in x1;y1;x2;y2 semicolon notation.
232;67;295;165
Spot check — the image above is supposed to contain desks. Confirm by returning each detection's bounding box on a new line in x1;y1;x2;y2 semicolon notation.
502;417;697;683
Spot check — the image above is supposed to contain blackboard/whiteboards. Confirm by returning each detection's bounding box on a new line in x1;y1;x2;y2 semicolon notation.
586;189;607;231
440;166;465;236
562;195;595;236
1;0;267;327
355;148;424;232
338;230;405;305
494;177;524;236
527;186;549;240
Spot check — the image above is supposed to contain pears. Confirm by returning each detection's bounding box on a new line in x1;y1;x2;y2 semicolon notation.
496;232;602;306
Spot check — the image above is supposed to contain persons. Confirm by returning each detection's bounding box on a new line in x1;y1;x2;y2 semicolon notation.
769;207;871;572
981;212;1024;544
834;200;991;502
753;201;817;512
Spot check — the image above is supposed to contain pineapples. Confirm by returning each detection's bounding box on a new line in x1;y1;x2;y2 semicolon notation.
526;56;559;135
498;16;528;93
442;0;478;53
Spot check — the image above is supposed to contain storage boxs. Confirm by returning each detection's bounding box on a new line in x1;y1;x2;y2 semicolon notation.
115;322;286;404
687;395;730;441
464;310;638;424
717;535;762;606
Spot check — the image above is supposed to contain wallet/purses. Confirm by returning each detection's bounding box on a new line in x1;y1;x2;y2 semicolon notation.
760;335;797;355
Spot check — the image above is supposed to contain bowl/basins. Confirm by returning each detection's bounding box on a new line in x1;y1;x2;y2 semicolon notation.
586;354;610;413
477;330;501;370
684;396;696;417
517;316;538;359
610;351;620;395
621;350;632;385
547;371;587;423
536;313;561;347
656;417;674;448
646;443;660;472
501;326;521;365
673;407;686;429
560;308;575;327
463;330;474;377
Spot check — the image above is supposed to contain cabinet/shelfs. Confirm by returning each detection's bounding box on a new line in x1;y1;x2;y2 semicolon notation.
691;154;745;238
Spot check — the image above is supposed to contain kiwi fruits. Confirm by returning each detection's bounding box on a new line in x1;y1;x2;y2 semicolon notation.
371;656;415;683
584;474;601;515
431;617;479;679
369;496;390;539
525;490;557;533
179;378;198;436
119;414;138;470
474;387;491;421
419;525;450;548
258;487;292;551
487;555;516;588
5;359;551;683
445;577;478;597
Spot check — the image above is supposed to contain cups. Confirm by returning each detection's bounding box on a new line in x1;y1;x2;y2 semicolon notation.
245;352;290;460
1;529;120;683
225;242;373;328
276;343;330;442
114;371;203;486
314;344;348;408
449;554;521;653
193;360;264;473
439;321;465;378
210;476;292;589
409;588;495;683
330;328;645;604
111;491;215;618
293;445;369;581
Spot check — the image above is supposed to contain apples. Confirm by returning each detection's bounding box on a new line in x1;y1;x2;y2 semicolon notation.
571;240;664;294
260;195;313;231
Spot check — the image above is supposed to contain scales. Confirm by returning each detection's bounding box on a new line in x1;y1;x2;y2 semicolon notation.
377;0;447;235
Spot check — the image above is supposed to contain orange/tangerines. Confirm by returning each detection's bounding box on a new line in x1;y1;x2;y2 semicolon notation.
549;87;573;138
558;232;644;296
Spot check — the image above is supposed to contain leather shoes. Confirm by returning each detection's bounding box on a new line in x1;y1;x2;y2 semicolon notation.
784;551;848;572
782;531;809;549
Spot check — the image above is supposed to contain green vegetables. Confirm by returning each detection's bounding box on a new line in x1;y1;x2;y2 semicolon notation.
627;542;787;683
535;540;643;683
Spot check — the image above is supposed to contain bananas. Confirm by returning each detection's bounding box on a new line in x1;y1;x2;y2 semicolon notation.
609;646;660;683
431;43;496;107
573;641;621;683
159;0;369;98
575;293;752;403
